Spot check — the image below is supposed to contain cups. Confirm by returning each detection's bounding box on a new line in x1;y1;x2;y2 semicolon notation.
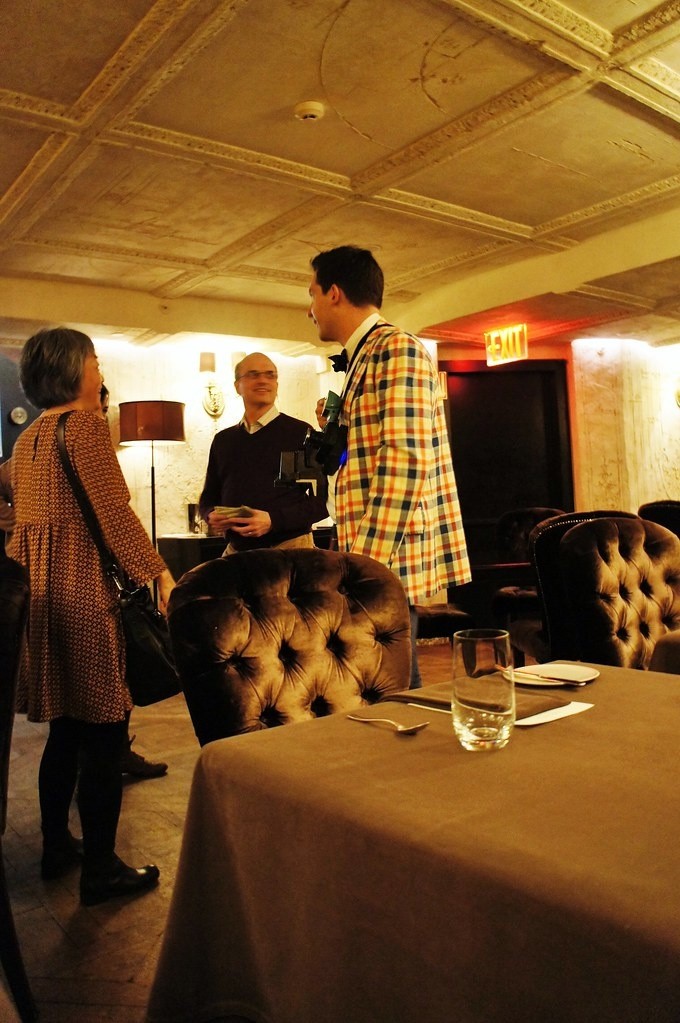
454;627;517;754
214;506;242;520
189;504;202;537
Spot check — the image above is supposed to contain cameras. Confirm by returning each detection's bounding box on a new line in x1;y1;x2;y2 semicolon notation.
304;389;347;474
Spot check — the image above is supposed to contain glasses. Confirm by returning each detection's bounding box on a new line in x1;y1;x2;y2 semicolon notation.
239;369;275;379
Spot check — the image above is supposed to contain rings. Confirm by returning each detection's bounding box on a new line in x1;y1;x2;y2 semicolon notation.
246;531;254;537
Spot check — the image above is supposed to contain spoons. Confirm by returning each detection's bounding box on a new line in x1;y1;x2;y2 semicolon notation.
346;709;430;736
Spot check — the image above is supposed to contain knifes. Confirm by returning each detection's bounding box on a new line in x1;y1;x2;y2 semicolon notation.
493;664;585;686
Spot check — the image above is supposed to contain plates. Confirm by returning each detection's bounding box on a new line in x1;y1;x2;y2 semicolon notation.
504;665;600;686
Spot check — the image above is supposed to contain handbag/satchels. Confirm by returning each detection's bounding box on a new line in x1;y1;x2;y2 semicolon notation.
118;584;182;707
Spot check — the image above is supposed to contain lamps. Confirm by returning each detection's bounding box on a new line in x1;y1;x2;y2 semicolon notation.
200;352;244;417
119;399;186;609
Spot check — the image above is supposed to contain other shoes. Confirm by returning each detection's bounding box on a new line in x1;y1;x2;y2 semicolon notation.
122;735;169;787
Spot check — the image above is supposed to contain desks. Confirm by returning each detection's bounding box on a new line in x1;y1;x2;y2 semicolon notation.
143;659;680;1023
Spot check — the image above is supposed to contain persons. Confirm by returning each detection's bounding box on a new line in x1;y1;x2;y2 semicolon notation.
307;243;474;690
1;325;176;909
197;352;329;558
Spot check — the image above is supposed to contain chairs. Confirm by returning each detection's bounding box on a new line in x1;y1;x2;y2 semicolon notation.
167;547;413;750
429;499;680;677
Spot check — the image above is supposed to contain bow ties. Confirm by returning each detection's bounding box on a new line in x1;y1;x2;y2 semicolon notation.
328;348;349;373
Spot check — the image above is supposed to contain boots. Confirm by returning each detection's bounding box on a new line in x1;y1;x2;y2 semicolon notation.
40;828;84;881
79;851;159;907
